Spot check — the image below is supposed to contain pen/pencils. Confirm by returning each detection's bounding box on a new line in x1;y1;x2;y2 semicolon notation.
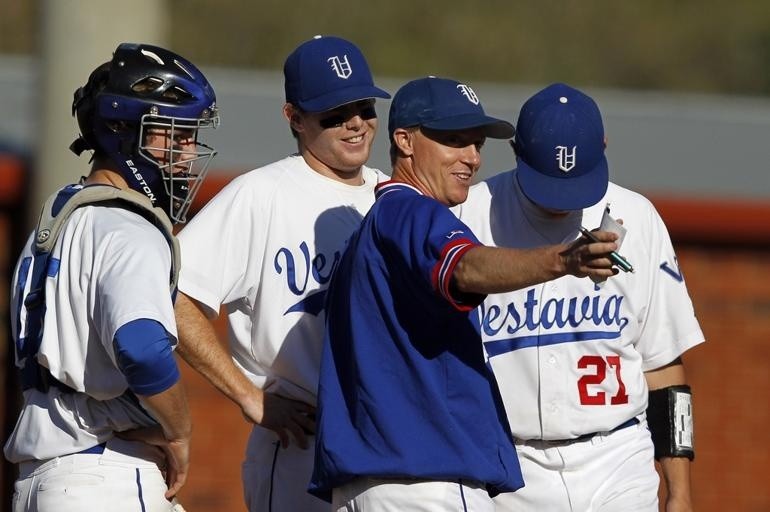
578;227;636;275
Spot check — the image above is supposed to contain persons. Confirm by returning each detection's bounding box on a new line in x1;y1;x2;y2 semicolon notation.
308;71;624;512
3;39;222;511
446;82;709;512
170;35;396;512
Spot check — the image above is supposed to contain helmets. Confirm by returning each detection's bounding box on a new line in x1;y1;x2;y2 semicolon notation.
68;42;219;139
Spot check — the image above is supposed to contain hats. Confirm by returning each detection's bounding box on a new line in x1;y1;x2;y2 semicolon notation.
515;82;610;212
283;33;392;115
387;74;516;140
